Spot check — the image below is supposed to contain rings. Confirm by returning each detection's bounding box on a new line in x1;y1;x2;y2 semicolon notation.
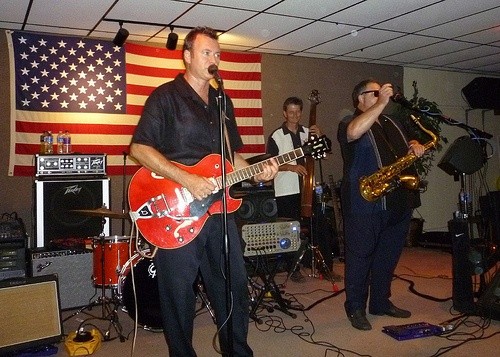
202;193;206;197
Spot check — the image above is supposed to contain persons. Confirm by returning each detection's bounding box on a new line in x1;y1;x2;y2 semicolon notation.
266;96;342;282
337;79;427;330
129;28;279;357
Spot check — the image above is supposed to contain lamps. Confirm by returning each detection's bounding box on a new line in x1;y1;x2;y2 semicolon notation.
166;26;178;50
113;21;130;47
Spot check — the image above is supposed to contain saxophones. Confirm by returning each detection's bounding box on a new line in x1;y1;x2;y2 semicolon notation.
358;113;440;202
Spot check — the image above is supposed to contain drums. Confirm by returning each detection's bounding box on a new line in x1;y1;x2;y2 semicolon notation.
118;249;198;333
91;236;141;289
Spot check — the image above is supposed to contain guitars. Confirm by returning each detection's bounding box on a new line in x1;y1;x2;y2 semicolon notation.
128;134;332;249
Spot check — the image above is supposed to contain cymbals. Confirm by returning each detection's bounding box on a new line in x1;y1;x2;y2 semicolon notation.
63;208;129;219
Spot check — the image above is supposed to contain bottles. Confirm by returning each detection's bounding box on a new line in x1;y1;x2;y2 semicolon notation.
40;131;49;155
314;182;324;204
63;130;71;154
57;131;65;154
44;131;53;154
458;188;467;213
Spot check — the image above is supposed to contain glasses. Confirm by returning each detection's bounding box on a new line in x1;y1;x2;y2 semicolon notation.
361;90;379;97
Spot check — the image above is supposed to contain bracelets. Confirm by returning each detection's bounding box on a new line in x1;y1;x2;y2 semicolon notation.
251;175;259;186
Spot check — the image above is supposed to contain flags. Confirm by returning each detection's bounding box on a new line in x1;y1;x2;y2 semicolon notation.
5;30;266;177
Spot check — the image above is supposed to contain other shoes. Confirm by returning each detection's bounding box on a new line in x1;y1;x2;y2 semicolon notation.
287;270;306;282
323;271;344;282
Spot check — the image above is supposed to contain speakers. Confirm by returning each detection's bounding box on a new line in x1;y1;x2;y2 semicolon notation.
436;136;487;177
34;177;111;249
0;272;64;357
462;77;500;109
447;217;500;316
26;246;111;306
233;190;278;239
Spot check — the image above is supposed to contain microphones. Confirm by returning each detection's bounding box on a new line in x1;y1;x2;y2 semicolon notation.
394;93;420;112
208;64;224;86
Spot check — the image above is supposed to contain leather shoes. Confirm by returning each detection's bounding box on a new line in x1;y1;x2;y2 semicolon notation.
346;309;371;331
369;301;412;318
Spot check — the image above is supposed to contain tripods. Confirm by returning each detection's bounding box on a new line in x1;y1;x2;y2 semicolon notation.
285;213;335;285
64;239;122;328
